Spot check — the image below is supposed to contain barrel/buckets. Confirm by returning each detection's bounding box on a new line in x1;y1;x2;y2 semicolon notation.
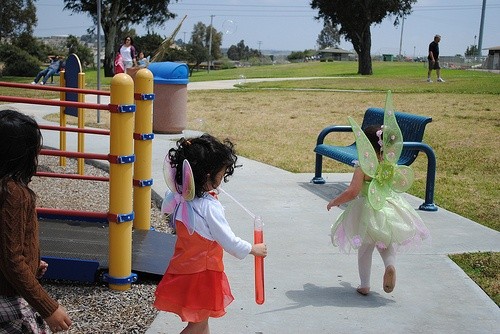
147;62;189;133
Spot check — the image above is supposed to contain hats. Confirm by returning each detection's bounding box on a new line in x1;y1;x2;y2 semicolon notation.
435;35;442;40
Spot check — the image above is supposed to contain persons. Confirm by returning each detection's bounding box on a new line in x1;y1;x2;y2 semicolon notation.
304;54;320;62
327;89;429;296
426;35;444;82
32;55;65;85
153;133;268;334
116;36;149;72
0;110;72;334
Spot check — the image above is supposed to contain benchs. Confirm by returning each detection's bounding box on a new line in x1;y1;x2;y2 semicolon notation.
310;106;438;214
42;62;65;84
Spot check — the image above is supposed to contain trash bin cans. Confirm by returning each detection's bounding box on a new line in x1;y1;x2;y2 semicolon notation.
145;61;190;135
381;53;394;62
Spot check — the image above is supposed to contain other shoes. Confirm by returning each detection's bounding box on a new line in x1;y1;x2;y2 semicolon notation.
382;265;395;294
357;285;370;294
436;78;445;83
426;79;433;83
40;82;43;85
32;81;36;84
